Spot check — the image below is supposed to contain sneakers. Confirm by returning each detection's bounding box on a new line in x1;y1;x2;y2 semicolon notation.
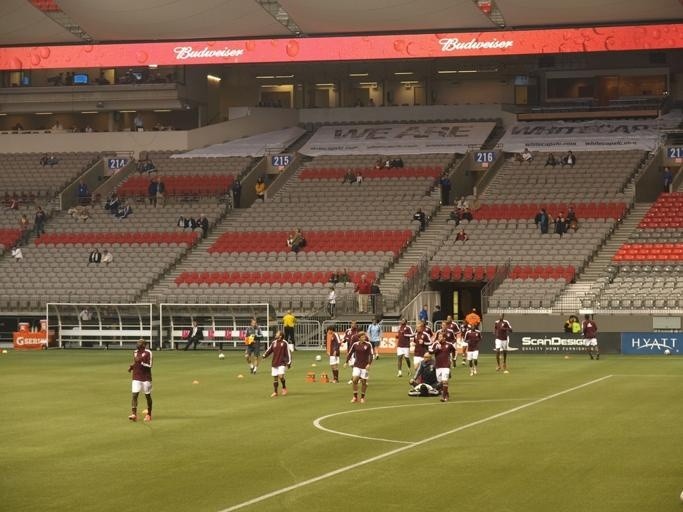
495;366;507;371
397;373;402;377
251;366;256;374
282;386;287;396
270;392;278;397
329;379;339;383
440;392;449;402
348;378;362;384
351;396;366;404
143;414;151;422
469;368;477;376
128;414;136;422
376;354;379;359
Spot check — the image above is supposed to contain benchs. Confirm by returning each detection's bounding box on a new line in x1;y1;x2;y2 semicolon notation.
168;340;270;349
56;339;152;350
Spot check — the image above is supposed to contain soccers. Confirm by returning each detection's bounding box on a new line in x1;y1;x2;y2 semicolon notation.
219;353;224;358
664;349;671;355
315;355;321;361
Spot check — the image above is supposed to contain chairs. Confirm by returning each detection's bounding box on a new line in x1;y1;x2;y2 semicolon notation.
0;148;683;312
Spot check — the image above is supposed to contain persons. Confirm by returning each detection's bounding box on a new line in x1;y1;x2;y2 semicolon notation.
515;146;576;168
89;248;101;264
101;249;114;263
437;167;481;244
414;208;425;232
177;214;208;235
255;176;266;201
537;206;580;235
133;111;177;131
8;196;46;261
67;183;90;222
367;98;376;107
286;228;305;252
79;306;93;322
374;156;403;169
184;321;204;351
45;63;174;85
341;168;364;185
128;338;153;421
241;269;513;403
664;166;672;193
14;121;95;132
353;97;363;107
255;99;284;109
39;152;58;168
234;179;241;209
105;159;165;219
564;315;600;360
